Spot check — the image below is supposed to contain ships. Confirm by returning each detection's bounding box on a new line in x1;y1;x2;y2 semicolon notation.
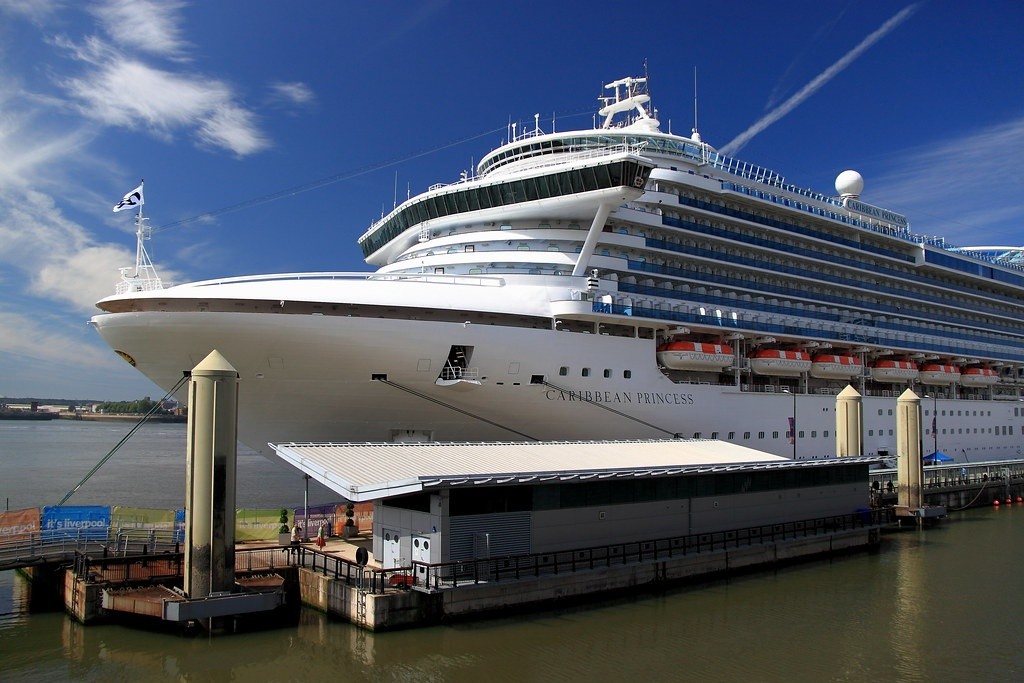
86;51;1023;511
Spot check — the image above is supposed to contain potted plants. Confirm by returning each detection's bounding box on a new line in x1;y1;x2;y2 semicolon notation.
342;503;357;537
278;506;291;545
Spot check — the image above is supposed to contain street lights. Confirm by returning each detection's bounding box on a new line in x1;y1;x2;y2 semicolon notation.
923;393;937;486
781;389;797;460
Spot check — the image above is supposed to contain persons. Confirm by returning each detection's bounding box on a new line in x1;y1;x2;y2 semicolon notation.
962;468;966;478
316;523;326;550
291;523;301;554
887;480;894;493
982;474;989;482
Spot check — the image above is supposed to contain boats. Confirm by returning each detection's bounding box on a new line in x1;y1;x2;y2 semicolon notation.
656;340;734;372
747;348;812;379
918;363;962;387
960;367;998;387
809;354;863;380
864;359;919;383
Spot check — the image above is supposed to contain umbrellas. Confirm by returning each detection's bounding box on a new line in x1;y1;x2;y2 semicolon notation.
923;452;954;462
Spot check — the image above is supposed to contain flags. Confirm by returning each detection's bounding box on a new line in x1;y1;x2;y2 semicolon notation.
931;417;936;438
113;185;144;212
